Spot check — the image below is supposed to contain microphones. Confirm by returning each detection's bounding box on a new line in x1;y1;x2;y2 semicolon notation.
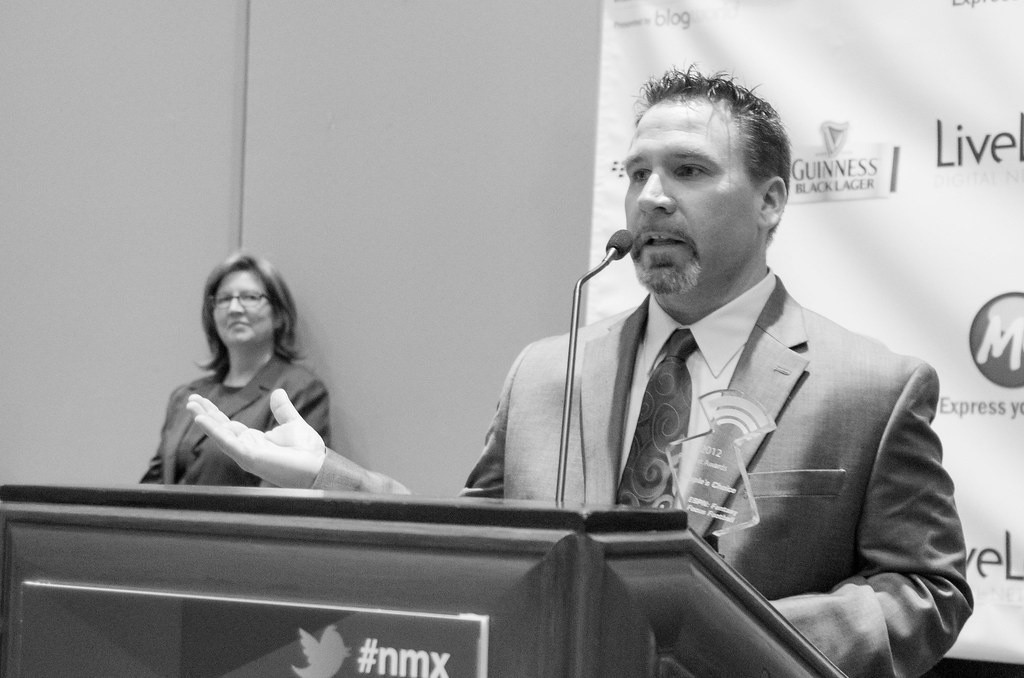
557;229;632;503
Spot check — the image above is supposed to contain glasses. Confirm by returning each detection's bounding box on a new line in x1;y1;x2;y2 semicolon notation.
210;293;266;310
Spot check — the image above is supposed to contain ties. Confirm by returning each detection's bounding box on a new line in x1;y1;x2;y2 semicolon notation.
616;326;697;509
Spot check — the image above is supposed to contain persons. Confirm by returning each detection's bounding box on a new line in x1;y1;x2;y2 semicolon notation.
186;72;976;678
137;250;330;487
